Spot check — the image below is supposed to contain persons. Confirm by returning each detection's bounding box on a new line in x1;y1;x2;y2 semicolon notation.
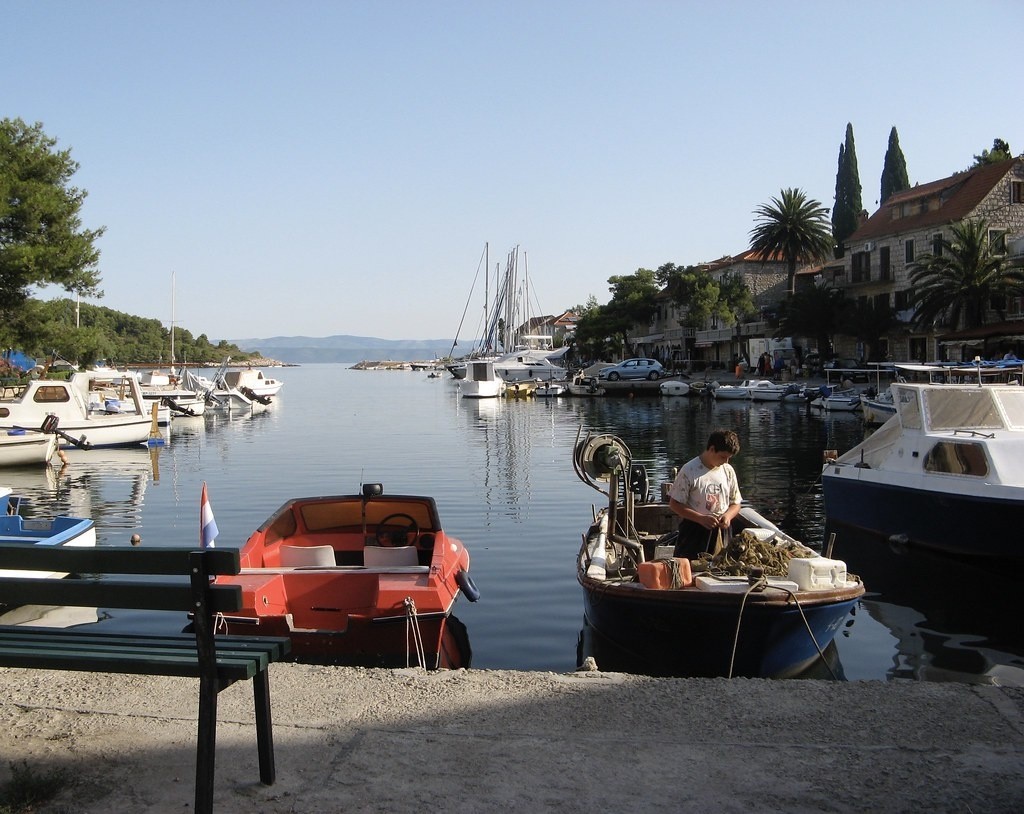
757;352;773;377
775;357;784;373
789;356;796;374
705;356;713;381
670;428;743;558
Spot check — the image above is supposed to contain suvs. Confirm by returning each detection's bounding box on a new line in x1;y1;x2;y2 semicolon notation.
598;358;665;382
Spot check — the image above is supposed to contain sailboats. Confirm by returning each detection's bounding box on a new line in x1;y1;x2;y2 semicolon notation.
443;242;570;382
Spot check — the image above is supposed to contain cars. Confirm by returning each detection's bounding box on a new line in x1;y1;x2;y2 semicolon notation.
820;358;873;383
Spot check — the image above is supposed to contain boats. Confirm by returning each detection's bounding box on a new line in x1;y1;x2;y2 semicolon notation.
820;356;1023;560
0;269;285;468
458;361;507;398
567;368;606;396
0;604;115;628
180;613;473;669
187;468;481;650
50;443;153;465
506;381;538;398
573;422;866;680
0;492;97;581
0;462;56;492
535;383;564;395
577;613;847;682
660;380;690;397
688;359;1024;428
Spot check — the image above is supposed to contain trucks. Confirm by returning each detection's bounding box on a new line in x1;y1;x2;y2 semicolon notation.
749;336;800;374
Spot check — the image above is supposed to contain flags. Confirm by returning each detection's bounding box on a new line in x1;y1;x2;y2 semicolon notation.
202;484;219;580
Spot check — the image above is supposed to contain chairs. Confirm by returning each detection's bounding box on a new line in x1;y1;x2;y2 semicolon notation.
654;545;675;562
363;546;420;566
279;544;338;568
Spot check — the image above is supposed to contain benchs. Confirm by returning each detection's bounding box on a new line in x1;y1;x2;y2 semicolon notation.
0;541;293;814
